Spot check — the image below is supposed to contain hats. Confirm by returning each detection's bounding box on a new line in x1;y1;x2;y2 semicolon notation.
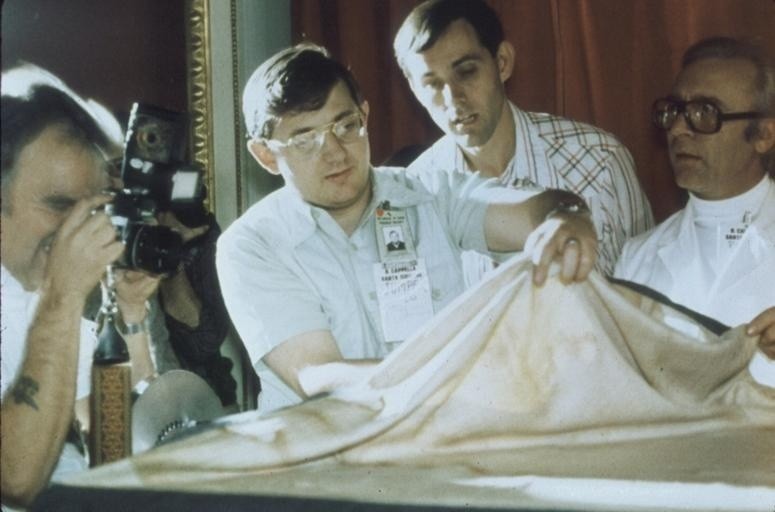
131;369;223;455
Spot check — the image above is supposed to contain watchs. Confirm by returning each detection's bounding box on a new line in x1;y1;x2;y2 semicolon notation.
543;200;592;222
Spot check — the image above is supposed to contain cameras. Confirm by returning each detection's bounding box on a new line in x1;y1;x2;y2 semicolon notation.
88;187;182;277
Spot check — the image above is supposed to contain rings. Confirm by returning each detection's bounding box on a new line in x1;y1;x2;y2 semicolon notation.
561;235;580;251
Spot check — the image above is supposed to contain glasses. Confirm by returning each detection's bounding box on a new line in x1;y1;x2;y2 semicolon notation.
258;113;361;160
650;98;769;134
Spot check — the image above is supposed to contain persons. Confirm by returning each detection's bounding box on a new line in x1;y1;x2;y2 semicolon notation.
387;230;405;250
392;0;654;291
1;58;241;512
612;36;775;388
213;43;599;409
